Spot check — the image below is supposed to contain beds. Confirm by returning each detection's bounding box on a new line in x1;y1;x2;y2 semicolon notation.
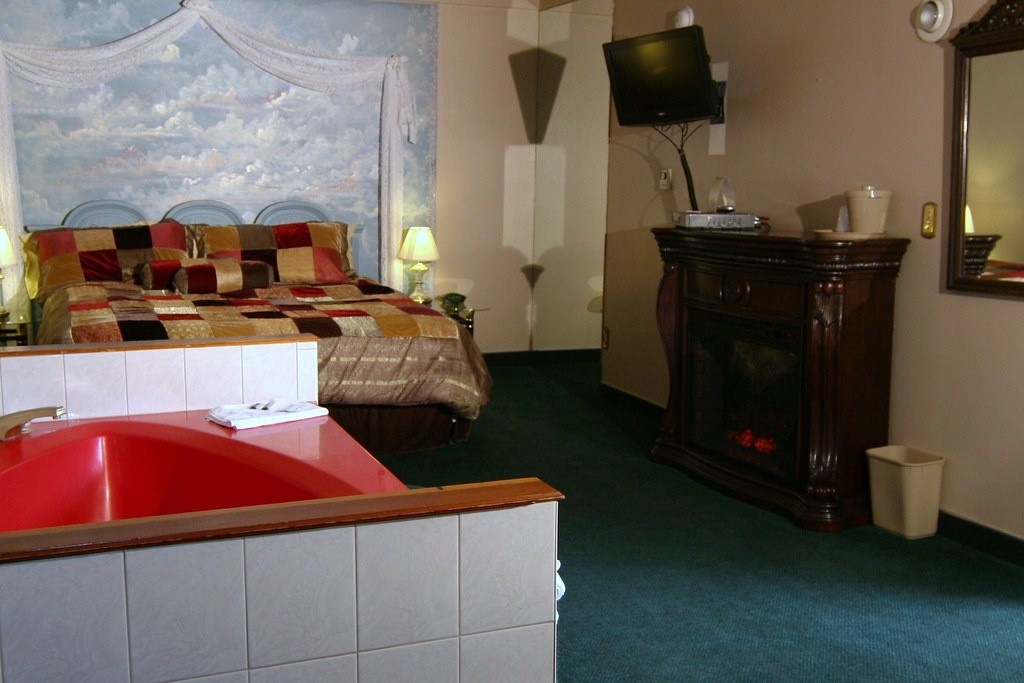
19;199;494;456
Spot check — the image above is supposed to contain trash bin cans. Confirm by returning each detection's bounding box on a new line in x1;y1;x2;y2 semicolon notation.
864;444;949;540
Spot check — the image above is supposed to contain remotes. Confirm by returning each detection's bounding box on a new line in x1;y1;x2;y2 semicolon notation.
716;205;735;213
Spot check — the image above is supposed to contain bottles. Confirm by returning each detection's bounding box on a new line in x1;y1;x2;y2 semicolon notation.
845;184;892;235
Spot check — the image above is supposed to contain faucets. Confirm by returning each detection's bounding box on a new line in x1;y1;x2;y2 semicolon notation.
0;406;67;441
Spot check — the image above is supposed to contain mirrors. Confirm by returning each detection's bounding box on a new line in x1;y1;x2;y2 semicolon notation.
946;0;1024;301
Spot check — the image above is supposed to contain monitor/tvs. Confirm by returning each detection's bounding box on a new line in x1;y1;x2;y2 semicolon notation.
602;25;716;128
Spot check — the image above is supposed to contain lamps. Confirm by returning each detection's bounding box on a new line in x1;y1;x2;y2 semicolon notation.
397;226;440;308
914;0;954;44
0;226;19;318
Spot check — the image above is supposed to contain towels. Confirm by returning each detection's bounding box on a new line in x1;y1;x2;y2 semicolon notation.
205;395;329;429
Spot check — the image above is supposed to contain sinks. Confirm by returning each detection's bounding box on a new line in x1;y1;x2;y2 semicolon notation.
0;420;364;534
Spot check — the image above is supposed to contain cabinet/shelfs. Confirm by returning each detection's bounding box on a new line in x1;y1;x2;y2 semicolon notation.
645;225;912;535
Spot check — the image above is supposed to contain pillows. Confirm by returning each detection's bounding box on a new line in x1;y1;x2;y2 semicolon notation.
19;220;357;309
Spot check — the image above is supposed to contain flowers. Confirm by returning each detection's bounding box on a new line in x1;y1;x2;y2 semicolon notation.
725;428;777;456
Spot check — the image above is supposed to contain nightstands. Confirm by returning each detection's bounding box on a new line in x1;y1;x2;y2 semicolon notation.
0;310;34;346
